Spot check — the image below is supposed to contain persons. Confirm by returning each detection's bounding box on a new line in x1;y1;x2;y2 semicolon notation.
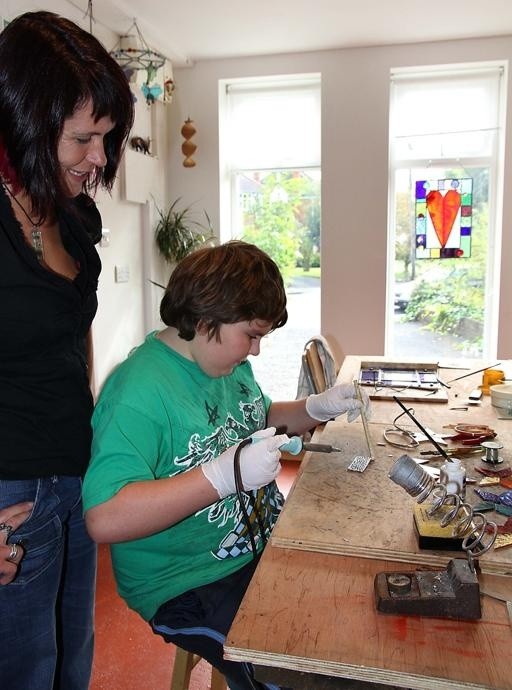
83;240;370;690
0;11;135;690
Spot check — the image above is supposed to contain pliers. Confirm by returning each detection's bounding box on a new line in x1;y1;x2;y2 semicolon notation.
451;432;497;445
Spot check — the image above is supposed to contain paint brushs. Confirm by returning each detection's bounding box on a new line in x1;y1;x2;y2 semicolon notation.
393;396;454;463
446;363;501;385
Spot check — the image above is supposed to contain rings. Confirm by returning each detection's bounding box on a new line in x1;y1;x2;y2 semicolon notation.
10;543;18;558
0;522;14;537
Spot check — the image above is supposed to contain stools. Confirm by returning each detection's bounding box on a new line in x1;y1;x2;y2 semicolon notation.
170;644;229;689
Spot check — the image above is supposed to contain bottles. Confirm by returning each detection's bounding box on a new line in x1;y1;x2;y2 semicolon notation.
482;369;505;396
440;458;466;500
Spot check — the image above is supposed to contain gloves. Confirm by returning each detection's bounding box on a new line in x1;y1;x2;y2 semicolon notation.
200;427;290;500
305;381;373;424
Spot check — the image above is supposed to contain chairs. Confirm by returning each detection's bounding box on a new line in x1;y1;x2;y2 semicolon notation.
302;337;336;396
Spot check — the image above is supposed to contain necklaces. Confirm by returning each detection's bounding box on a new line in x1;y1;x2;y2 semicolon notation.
2;183;47;262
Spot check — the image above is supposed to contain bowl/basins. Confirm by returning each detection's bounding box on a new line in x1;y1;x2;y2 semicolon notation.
489;384;512;419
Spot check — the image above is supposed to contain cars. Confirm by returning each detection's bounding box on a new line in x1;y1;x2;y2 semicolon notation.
394;266;485;311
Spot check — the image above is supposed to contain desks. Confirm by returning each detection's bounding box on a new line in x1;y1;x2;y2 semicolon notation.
223;353;512;689
